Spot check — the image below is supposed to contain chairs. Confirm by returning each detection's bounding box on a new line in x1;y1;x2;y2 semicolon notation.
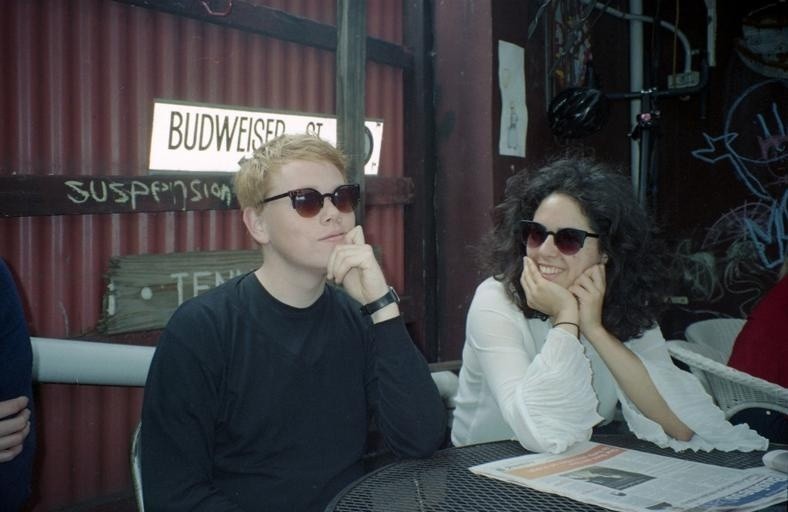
668;339;788;422
684;319;748;363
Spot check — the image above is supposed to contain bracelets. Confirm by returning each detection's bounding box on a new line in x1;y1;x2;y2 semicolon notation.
553;322;579;329
359;285;403;317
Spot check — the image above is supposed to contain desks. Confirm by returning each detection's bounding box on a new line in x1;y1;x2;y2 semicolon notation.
326;434;788;512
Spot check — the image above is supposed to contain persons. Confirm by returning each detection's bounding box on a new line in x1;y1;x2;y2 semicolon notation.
717;268;788;445
0;258;38;512
448;156;769;512
139;134;449;512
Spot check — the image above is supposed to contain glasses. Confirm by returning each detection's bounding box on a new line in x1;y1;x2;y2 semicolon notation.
513;215;604;255
257;182;362;218
